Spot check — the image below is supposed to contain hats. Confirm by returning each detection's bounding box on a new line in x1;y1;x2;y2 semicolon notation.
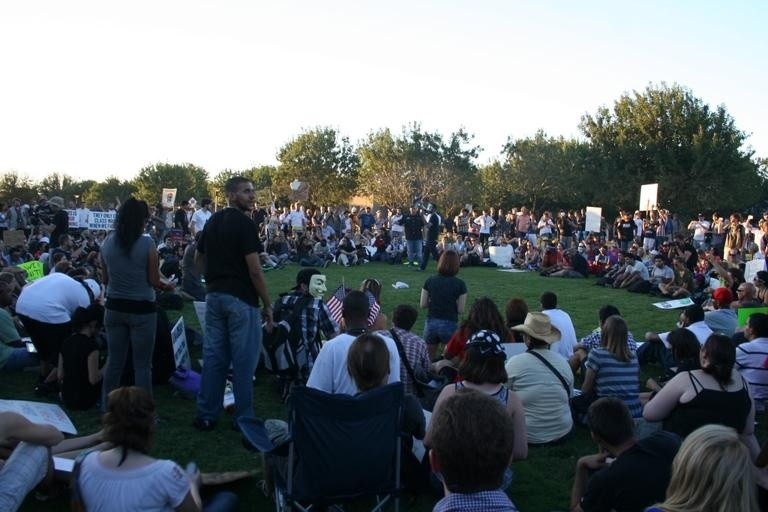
713;288;732;305
510;312;562;344
290;269;320;290
342;291;369;331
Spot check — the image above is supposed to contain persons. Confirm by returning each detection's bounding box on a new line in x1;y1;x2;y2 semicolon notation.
194;176;272;430
79;387;239;511
572;396;683;510
0;412;65;511
0;198;768;462
429;389;517;510
756;446;768;491
645;424;759;510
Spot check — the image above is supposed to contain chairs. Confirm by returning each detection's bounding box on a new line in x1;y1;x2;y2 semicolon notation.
256;322;320;403
267;382;404;511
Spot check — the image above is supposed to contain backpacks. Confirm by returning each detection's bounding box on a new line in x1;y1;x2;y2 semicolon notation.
260;294;316;384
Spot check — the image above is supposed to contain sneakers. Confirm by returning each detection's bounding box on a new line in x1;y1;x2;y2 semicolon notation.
191;419;217;430
223;385;235;408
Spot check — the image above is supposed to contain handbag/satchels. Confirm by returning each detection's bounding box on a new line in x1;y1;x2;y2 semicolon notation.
413;369;448;405
